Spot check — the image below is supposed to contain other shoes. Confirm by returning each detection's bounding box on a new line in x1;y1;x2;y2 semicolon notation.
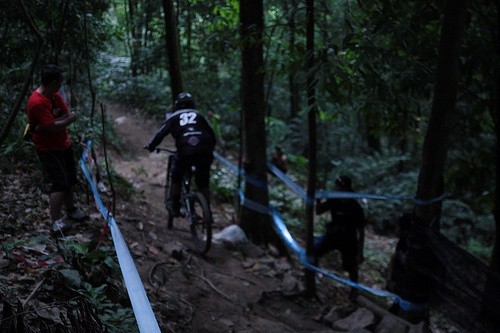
50;216;69;234
166;201;180;217
66;207;89;222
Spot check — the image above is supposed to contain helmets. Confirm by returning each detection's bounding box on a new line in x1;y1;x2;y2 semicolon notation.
175;92;193;102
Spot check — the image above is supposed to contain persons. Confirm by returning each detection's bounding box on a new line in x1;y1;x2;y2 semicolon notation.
146;92;218;225
267;145;289;184
300;174;365;301
25;66;90;236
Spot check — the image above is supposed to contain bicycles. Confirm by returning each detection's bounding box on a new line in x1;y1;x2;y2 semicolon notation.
145;145;213;253
